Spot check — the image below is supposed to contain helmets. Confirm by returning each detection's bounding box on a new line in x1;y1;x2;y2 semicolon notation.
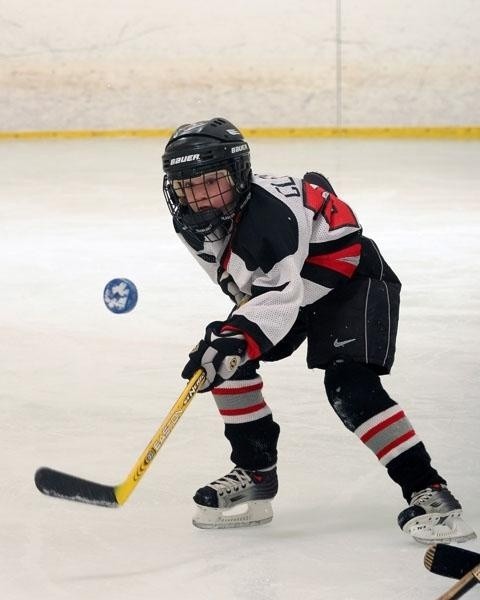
161;118;253;243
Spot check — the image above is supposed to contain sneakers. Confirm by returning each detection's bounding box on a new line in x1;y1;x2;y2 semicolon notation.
193;465;278;509
397;487;462;532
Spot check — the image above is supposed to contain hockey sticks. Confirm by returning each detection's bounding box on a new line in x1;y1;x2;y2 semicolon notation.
36;293;251;507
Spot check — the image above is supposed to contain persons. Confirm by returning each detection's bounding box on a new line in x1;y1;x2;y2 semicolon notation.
162;118;462;532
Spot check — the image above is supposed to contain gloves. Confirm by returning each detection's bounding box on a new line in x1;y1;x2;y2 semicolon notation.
181;321;248;393
